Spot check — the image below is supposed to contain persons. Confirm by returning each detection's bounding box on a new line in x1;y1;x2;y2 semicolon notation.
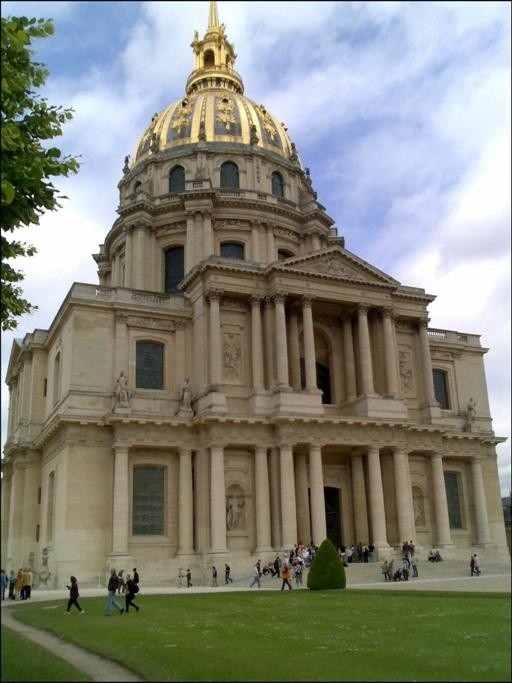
381;553;419;583
339;541;375;563
250;540;317;591
178;567;192;588
212;566;217;587
398;360;415;393
177;375;195;411
226;492;245;533
224;342;241;375
224;563;233;584
0;566;141;616
413;496;427;527
401;540;415;558
428;550;443;563
469;554;481;576
112;368;133;408
466;395;478;426
